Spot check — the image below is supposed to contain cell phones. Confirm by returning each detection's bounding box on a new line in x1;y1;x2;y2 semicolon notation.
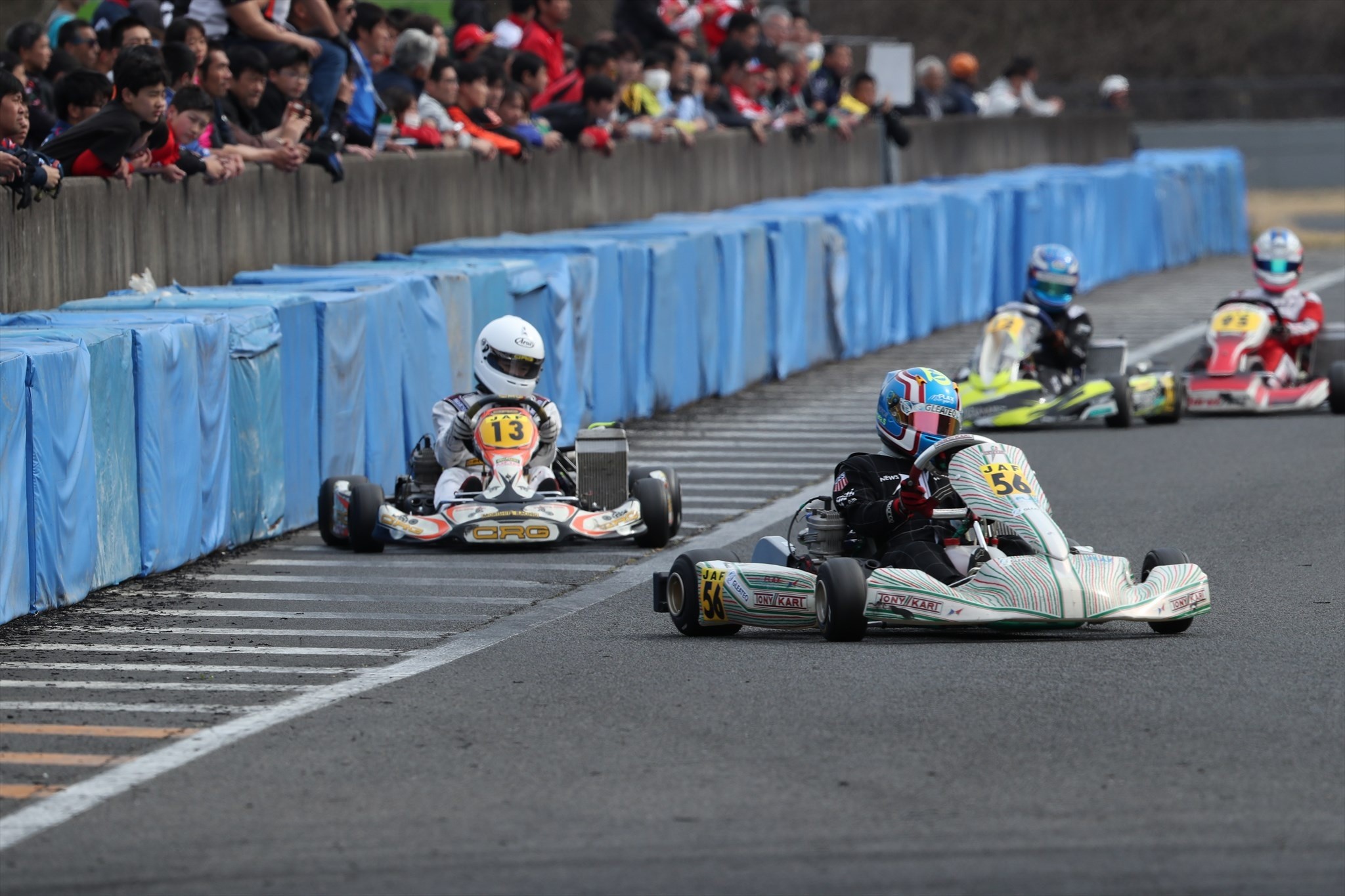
289;98;306;120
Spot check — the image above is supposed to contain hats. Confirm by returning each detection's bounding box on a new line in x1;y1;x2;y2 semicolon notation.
1097;75;1129;100
491;18;522;49
454;23;496;52
949;52;978;79
394;28;433;73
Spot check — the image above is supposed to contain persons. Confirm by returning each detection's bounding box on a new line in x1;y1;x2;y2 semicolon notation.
1099;75;1140;151
989;243;1094;396
1185;226;1325;389
0;0;1063;190
433;315;563;513
833;366;1080;588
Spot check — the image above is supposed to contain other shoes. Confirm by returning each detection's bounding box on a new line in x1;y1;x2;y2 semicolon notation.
537;476;559;497
455;476;484;504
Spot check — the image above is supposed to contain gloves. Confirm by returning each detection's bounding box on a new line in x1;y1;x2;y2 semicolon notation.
894;478;941;518
446;411;475;452
539;417;558;444
332;29;350;63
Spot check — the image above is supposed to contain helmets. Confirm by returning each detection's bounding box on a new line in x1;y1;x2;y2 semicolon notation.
475;315;545;396
875;367;959;458
1025;242;1081;308
1251;226;1305;293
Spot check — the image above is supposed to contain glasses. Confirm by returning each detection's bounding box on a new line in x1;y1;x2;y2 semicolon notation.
345;3;359;14
80;39;96;47
